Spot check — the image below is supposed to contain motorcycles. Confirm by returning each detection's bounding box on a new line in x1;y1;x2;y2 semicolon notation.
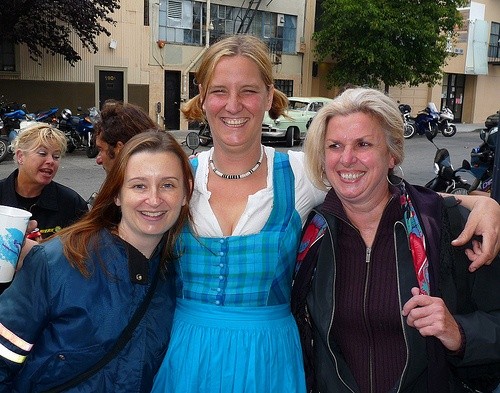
0;94;98;164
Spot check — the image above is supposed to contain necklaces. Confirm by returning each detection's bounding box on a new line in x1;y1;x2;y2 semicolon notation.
209;144;264;179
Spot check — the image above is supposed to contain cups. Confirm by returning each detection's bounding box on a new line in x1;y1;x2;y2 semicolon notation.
0;205;32;283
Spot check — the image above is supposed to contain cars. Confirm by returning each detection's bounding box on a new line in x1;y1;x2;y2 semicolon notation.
261;96;334;147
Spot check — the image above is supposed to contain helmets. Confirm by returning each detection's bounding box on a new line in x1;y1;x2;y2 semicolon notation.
60;109;72;120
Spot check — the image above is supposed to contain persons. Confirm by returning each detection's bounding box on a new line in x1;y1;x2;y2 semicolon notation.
0;128;194;393
0;121;89;294
288;88;500;393
94;98;158;176
14;36;499;393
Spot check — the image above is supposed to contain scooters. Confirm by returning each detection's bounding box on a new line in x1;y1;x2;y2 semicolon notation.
396;99;500;195
197;120;214;147
